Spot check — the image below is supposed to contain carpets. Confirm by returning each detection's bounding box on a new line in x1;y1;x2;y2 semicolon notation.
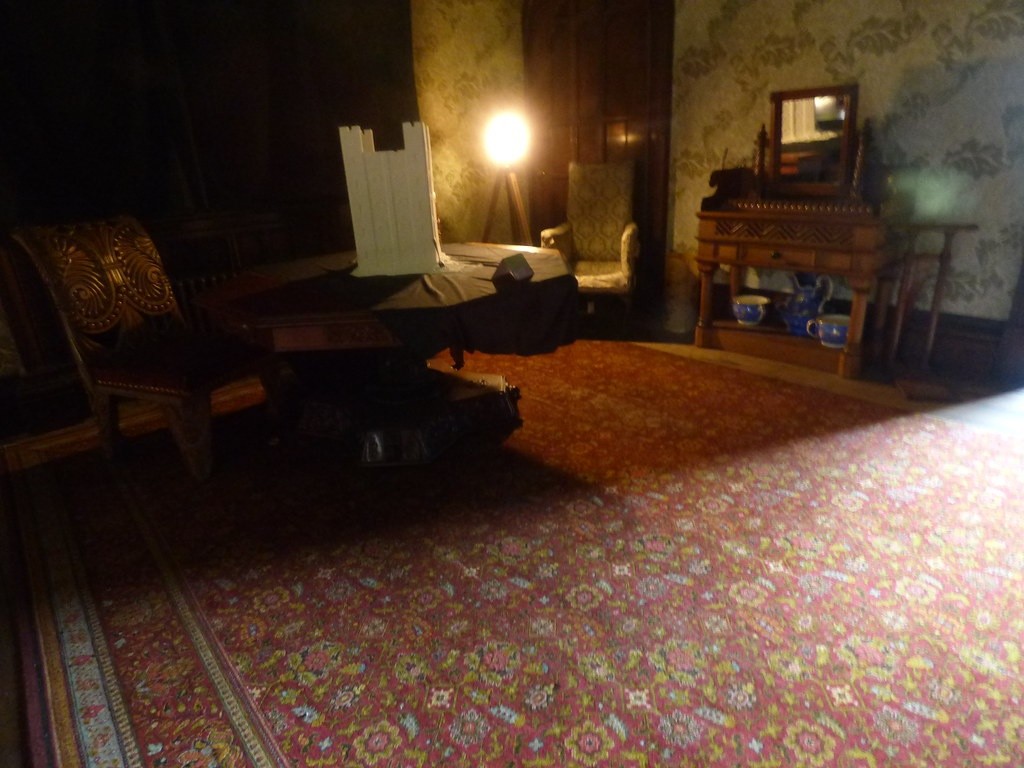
0;339;1024;768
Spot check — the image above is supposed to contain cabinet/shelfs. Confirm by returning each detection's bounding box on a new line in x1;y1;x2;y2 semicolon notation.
694;211;904;380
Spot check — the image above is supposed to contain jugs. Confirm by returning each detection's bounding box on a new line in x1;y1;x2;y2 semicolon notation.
784;273;833;337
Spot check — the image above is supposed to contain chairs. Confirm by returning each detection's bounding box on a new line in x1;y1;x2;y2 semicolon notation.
10;214;279;487
541;163;640;338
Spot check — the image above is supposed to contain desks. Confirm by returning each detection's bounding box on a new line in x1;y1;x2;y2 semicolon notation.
190;241;577;470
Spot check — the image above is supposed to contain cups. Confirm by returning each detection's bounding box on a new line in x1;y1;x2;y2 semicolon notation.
733;296;771;325
807;314;851;349
